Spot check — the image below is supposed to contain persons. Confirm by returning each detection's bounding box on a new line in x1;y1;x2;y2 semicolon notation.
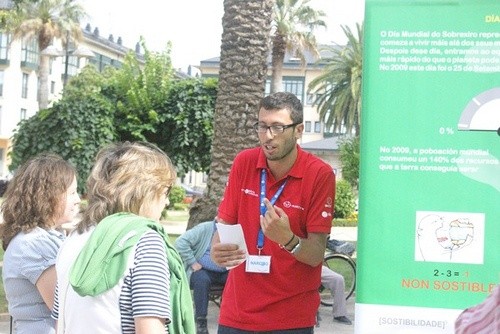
51;141;178;334
173;216;229;334
317;265;352;325
0;152;81;334
210;92;336;334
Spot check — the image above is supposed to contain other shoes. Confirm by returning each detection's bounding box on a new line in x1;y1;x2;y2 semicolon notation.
195;318;209;334
334;316;352;326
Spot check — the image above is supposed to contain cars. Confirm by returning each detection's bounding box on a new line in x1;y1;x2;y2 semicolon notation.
181;183;202;203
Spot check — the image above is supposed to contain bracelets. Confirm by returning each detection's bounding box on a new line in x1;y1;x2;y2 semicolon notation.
283;233;295;248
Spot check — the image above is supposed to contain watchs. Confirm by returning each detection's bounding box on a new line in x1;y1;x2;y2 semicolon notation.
284;235;303;256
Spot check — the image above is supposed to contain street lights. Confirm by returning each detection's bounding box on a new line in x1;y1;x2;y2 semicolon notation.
40;31;97;101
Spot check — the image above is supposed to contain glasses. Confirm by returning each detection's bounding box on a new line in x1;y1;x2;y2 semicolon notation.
160;184;173;197
254;121;303;134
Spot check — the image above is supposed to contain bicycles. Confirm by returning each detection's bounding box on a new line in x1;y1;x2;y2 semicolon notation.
318;251;357;307
